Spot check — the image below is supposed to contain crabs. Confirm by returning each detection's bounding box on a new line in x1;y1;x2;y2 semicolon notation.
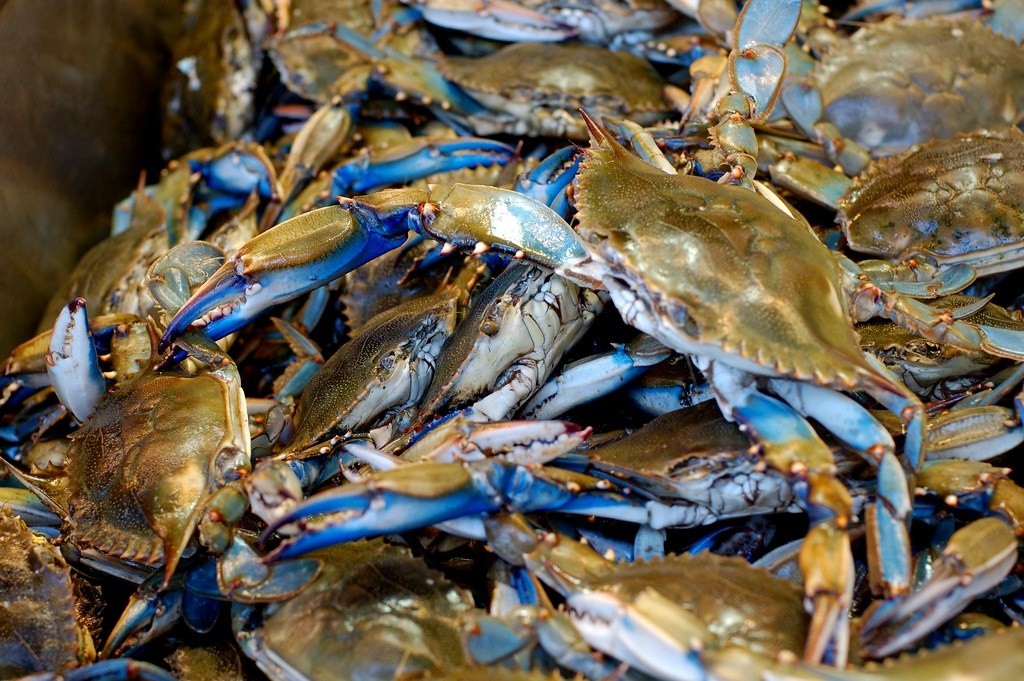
0;1;1024;681
